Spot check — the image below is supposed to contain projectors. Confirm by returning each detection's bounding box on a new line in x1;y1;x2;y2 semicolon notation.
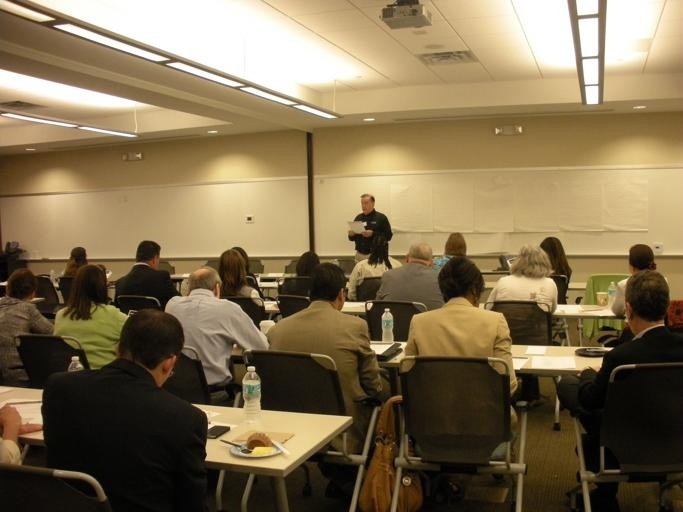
380;3;432;30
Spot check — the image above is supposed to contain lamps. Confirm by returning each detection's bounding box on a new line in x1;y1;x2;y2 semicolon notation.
0;0;342;119
567;0;607;104
1;107;142;139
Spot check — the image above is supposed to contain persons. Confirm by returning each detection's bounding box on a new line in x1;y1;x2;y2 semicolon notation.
611;244;668;347
1;232;466;512
555;269;682;510
400;255;520;479
348;193;392;264
539;237;572;304
484;244;557;410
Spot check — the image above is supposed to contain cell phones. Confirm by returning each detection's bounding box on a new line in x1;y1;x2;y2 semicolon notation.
205;424;229;438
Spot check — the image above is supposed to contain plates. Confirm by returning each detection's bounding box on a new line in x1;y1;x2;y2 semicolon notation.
575;347;608;357
230;441;281;458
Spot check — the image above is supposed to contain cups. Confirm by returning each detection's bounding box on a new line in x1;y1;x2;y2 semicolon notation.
259;320;275;333
595;291;608;306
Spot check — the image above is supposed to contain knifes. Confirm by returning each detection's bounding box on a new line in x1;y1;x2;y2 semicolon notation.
220;440;252;454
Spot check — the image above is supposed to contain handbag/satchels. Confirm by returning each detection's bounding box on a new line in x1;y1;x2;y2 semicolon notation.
356;395;425;512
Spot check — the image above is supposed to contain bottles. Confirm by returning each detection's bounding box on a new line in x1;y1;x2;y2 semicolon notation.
241;366;264;424
67;356;84;372
608;282;616;305
381;308;395;343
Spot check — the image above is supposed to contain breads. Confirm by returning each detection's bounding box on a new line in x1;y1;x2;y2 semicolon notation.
246;433;271;450
